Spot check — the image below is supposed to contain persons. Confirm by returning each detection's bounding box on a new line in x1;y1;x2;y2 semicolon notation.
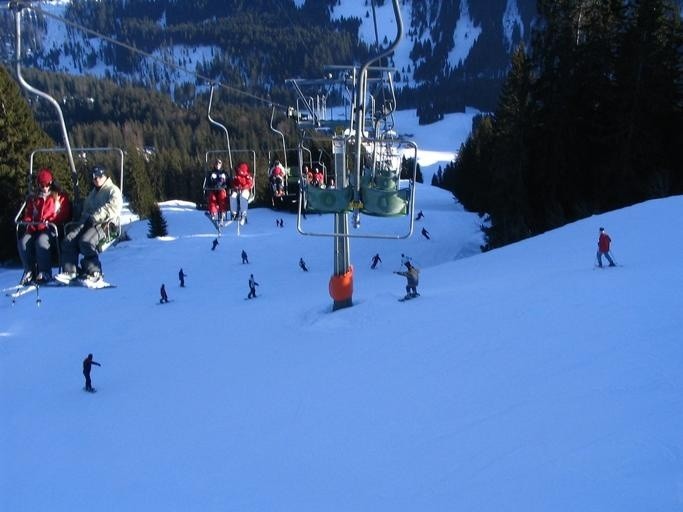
207;159;230;227
179;268;187;287
160;284;169;303
228;163;253;226
269;159;338;228
55;166;123;288
299;257;309;271
248;274;259;298
84;354;100;391
370;209;432;299
211;238;219;250
597;227;616;267
18;170;71;285
242;250;248;264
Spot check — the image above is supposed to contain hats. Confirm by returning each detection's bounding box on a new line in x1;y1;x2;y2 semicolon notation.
237;163;247;173
37;169;53;184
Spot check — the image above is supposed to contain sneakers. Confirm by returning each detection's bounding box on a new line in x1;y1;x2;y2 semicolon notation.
20;270;51;286
53;270;104;288
211;212;245;221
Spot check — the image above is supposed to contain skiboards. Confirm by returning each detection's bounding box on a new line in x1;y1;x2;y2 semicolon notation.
2;276;56;298
204;211;222;237
224;220;240;237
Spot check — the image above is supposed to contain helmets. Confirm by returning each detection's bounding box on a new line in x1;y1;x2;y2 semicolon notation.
89;164;106;177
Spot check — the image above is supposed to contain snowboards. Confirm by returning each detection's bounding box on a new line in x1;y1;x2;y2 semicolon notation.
43;276;118;289
